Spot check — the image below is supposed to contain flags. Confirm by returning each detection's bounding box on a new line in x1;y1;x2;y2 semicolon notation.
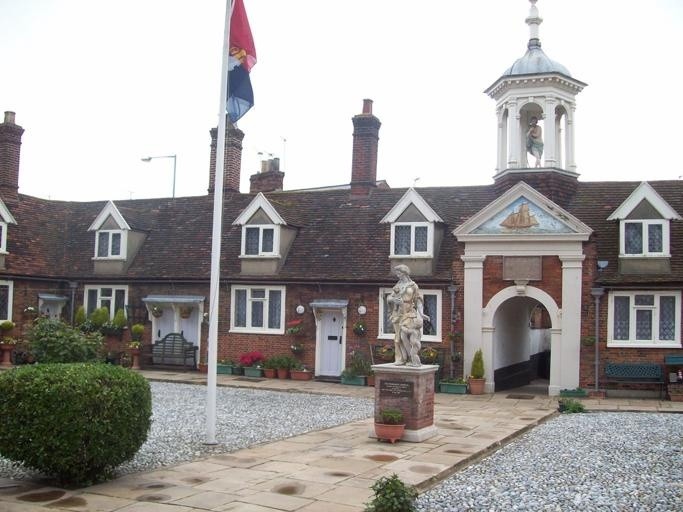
226;0;258;123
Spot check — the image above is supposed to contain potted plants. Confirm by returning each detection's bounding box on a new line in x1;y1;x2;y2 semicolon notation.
127;325;143;370
366;408;407;445
352;320;368;337
439;377;467;394
284;323;305;337
199;350;313;382
22;304;38;320
0;336;18;366
466;348;486;395
340;360;375;387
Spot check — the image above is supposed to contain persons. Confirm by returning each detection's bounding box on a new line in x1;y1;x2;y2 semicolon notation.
527;117;543;168
387;264;431;366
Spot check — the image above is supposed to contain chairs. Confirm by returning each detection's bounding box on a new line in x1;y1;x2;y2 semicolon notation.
139;332;195;372
601;362;663;401
